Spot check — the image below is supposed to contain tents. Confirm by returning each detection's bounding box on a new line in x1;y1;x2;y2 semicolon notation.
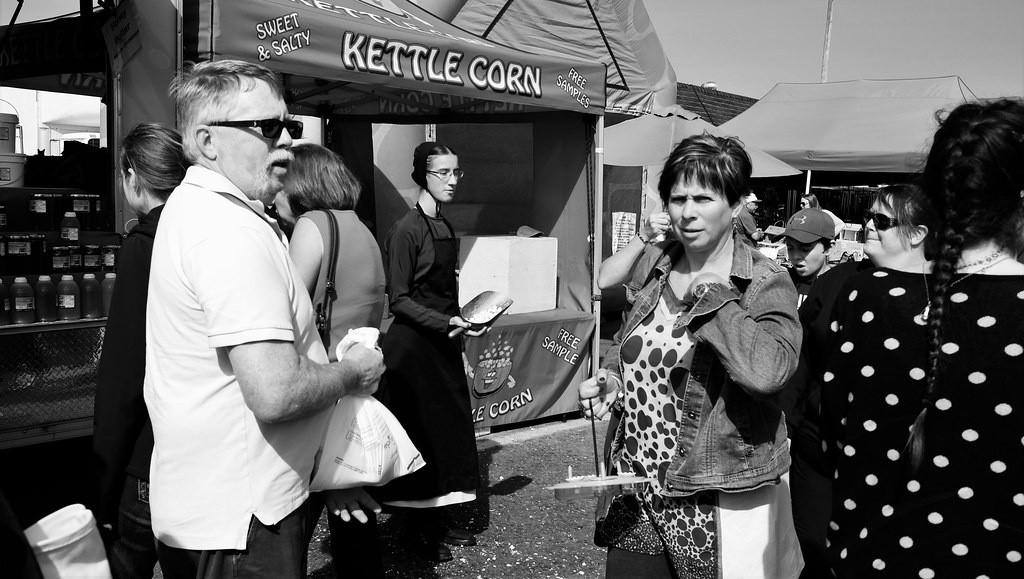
716;74;985;209
0;0;678;438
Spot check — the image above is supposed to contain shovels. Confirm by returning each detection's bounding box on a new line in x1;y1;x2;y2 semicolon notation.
447;291;513;338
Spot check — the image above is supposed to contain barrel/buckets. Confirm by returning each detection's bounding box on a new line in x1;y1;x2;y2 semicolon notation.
0;152;27;188
0;113;20;153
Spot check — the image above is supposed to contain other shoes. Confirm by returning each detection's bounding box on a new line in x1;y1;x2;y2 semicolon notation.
421;516;474;546
399;528;454;561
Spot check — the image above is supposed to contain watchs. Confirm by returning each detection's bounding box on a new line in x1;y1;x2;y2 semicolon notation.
693;282;716;304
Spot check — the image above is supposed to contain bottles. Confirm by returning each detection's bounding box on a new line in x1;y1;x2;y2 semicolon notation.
0;272;117;326
59;211;80;248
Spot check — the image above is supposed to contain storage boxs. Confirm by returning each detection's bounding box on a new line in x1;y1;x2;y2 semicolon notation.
458;235;560;314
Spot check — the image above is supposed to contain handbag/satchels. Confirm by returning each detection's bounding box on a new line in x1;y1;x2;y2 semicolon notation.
312;313;330;351
308;326;426;491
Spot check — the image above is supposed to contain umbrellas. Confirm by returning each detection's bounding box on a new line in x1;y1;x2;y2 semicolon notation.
603;104;803;212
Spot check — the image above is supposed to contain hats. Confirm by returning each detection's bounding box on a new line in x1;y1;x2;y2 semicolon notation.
744;192;763;205
781;208;835;244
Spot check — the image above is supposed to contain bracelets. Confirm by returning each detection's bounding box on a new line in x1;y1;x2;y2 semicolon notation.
637;232;648;244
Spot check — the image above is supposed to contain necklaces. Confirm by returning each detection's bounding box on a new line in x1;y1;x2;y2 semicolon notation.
956;245;1004;270
921;256;1010;320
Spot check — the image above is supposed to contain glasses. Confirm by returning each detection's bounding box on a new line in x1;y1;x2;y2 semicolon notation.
205;117;303;140
428;167;465;180
752;201;759;206
800;202;810;206
862;208;912;232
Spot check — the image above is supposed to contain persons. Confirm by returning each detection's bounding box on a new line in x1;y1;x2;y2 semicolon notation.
142;58;387;579
772;208;835;310
273;142;385;364
818;95;1024;579
89;121;202;579
380;142;492;563
735;192;763;249
799;193;845;239
577;129;803;579
782;183;930;579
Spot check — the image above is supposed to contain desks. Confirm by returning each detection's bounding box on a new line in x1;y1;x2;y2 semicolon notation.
463;307;597;428
